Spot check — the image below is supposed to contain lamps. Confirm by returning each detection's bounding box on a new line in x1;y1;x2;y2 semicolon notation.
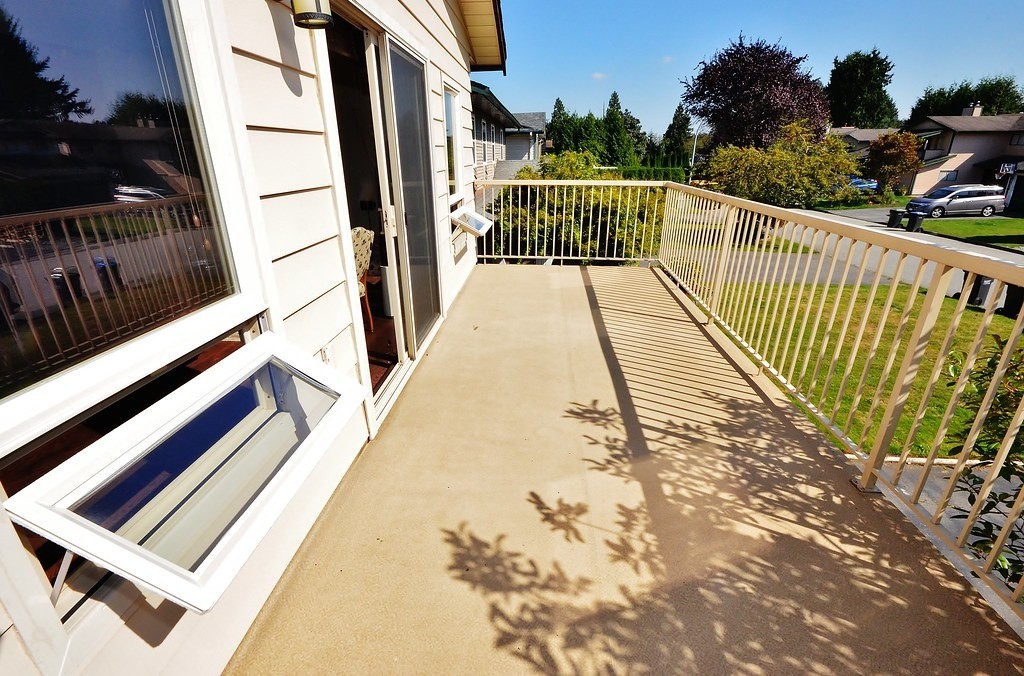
292;0;334;30
360;200;376;225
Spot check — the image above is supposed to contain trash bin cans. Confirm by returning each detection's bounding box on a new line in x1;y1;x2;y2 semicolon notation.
886;208;907;228
89;255;123;290
952;270;995;306
998;283;1024;315
906;211;927;233
45;265;82;307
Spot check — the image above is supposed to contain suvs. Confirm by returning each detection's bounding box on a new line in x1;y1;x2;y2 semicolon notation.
906;183;1006;219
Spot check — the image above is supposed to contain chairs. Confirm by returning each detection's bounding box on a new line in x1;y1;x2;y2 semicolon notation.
351;226;374;335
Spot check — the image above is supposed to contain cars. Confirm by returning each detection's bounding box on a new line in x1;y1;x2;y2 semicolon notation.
114;186;200;220
831;178;879;194
